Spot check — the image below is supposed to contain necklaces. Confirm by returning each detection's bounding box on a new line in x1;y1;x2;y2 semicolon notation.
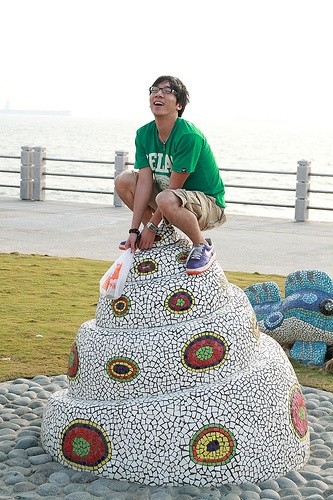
159;134;169;152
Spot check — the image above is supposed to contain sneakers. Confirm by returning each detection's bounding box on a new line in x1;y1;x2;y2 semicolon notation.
119;241;126;250
185;237;216;274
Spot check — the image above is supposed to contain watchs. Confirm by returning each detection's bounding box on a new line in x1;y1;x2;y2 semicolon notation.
146;222;158;232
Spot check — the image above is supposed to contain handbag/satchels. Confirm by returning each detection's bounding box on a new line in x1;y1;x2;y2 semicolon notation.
99;248;134;300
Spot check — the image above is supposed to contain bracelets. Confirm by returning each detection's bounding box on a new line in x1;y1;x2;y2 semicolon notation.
129;229;140;234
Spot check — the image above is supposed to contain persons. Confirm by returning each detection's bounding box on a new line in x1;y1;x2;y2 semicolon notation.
113;76;227;274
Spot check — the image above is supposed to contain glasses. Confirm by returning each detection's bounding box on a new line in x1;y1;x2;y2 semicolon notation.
149;86;178;96
